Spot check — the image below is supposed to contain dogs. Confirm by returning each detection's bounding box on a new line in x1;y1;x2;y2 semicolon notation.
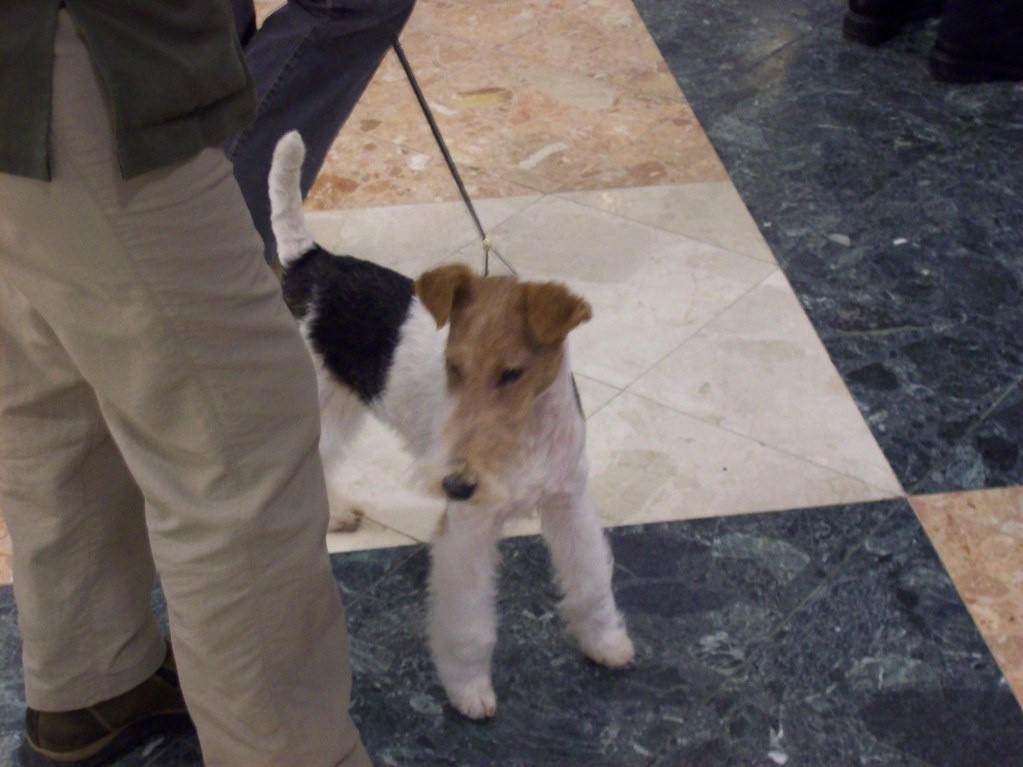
267;131;635;720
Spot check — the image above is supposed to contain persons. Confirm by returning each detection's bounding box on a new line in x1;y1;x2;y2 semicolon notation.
0;0;417;767
843;0;1023;84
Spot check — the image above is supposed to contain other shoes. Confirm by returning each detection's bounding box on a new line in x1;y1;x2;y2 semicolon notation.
23;632;188;767
844;0;942;38
928;23;1023;84
